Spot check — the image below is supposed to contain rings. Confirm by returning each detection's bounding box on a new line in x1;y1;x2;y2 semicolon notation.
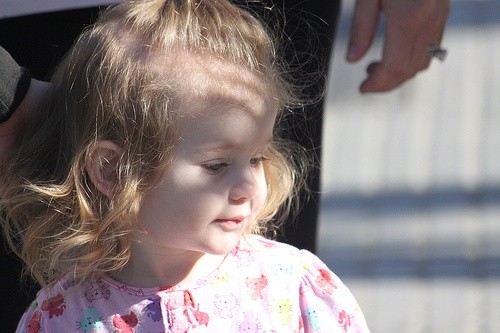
428;42;448;63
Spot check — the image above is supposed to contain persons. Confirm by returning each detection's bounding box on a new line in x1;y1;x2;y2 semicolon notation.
0;44;54;213
16;0;374;332
0;0;451;333
